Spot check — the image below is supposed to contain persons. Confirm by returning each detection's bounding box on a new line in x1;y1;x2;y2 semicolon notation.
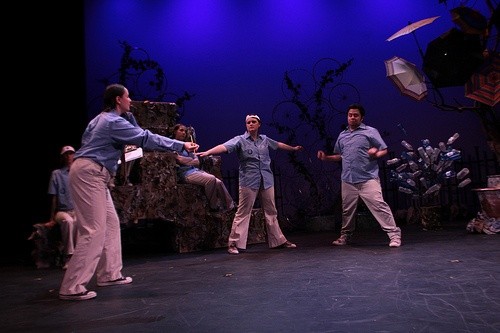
317;103;402;247
173;123;237;219
57;83;200;300
48;146;79;270
193;114;303;255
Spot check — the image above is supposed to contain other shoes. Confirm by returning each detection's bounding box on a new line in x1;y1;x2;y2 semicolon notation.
228;206;239;215
209;207;222;219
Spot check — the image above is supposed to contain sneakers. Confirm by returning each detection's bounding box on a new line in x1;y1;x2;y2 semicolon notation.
59;289;97;300
97;276;132;286
389;239;401;247
63;256;72;269
276;241;296;248
228;247;239;254
332;237;348;246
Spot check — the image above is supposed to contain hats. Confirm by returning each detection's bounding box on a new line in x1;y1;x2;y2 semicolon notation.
60;146;75;155
